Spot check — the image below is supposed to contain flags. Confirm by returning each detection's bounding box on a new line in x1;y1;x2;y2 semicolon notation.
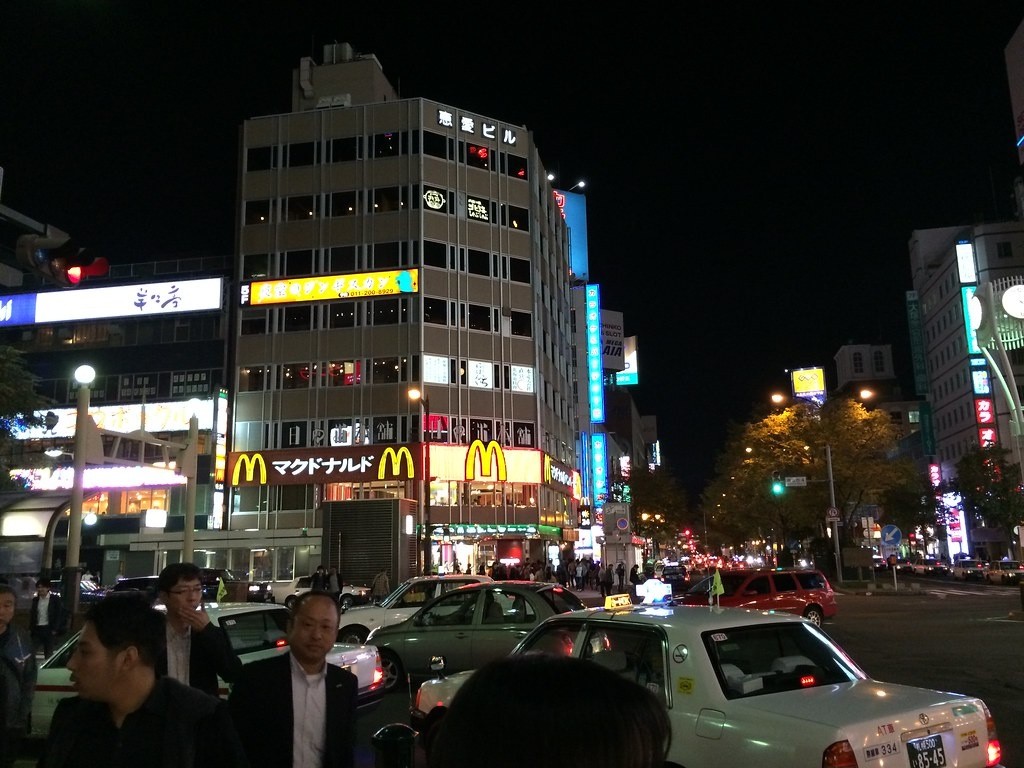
711;569;724;596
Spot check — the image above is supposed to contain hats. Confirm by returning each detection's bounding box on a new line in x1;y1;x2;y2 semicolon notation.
634;565;639;568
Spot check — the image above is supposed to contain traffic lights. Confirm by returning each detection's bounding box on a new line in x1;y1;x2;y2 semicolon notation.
771;470;784;497
16;233;112;289
685;530;691;536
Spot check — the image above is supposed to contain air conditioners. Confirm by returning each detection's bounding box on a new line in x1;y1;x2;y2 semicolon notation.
562;529;579;541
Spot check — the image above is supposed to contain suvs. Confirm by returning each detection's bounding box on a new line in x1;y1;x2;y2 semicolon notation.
953;559;987;582
891;559;913;575
912;558;948;578
873;558;886;570
336;573;515;646
985;561;1024;587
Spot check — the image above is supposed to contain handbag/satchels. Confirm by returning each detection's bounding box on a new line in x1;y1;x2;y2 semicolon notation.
615;568;618;574
371;585;374;593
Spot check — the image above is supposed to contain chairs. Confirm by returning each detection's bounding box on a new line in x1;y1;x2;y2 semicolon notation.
769;654;815;672
514;595;526;623
485;602;505;623
593;648;634;683
718;657;751;675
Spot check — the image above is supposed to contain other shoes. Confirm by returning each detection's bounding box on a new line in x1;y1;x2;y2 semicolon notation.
580;589;582;591
577;589;580;592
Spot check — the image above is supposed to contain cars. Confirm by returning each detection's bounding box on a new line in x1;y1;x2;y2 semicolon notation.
27;600;384;746
653;558;666;580
672;567;839;628
279;575;371;610
409;593;1003;768
660;566;692;590
361;582;612;699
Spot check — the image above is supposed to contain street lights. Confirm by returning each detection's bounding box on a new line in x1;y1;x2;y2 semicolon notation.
64;364;96;567
771;393;843;587
407;387;432;576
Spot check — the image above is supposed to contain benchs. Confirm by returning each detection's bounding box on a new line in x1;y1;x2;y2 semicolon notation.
230;629;287;649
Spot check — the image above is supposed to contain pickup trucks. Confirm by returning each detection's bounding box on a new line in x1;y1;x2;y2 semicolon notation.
113;567;274;604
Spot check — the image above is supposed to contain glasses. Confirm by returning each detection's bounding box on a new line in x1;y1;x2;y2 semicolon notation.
169;586;204;596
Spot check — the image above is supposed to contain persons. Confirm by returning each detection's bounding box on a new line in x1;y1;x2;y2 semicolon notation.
488;558;568;588
479;562;486;575
630;564;639;585
598;562;614;597
0;584;39;767
615;561;626;592
158;563;243;698
370;568;390;604
453;565;462;574
904;552;923;560
27;578;63;661
308;566;329;591
325;565;343;597
560;557;600;591
44;590;248;768
431;655;673;768
984;551;990;563
466;564;471;574
228;591;359;768
